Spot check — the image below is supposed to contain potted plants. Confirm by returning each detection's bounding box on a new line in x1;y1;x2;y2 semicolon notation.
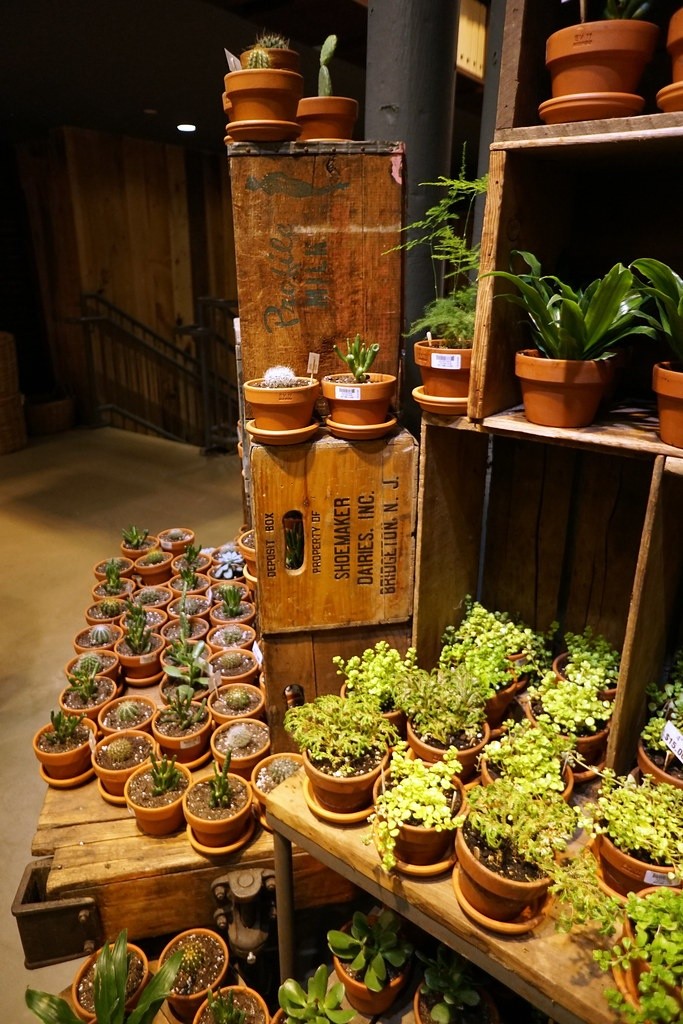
32;532;683;1024
223;0;683;447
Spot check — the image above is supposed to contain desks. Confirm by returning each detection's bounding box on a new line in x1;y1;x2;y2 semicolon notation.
267;766;613;1024
